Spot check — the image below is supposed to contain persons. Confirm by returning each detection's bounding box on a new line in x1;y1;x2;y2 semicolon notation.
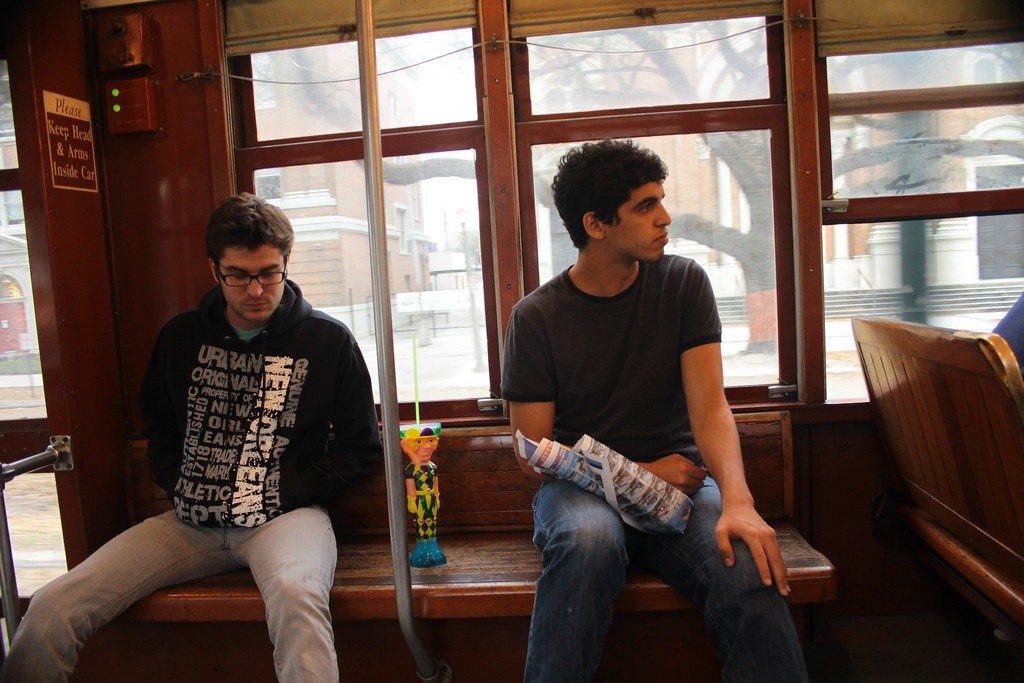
1;191;382;683
499;138;808;683
991;289;1024;381
401;424;447;566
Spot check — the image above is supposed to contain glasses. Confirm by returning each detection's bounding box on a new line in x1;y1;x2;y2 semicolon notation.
216;255;287;287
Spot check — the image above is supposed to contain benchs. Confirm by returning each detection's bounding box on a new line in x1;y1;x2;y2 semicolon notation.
852;314;1024;646
125;410;838;622
